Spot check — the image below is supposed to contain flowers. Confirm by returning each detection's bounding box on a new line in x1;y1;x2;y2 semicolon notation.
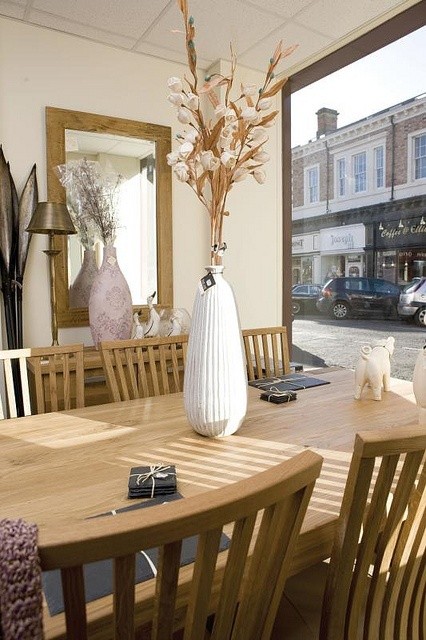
52;155;127;248
164;1;298;265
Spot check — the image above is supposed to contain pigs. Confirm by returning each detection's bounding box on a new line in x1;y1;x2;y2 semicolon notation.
352;335;395;401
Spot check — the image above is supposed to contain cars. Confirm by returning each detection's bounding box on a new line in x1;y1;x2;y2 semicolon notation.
398;276;426;326
291;285;324;314
316;277;402;319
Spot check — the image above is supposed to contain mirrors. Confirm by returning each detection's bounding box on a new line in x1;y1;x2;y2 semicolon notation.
45;106;173;326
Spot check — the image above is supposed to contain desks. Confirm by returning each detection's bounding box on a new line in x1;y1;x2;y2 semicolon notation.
0;369;425;638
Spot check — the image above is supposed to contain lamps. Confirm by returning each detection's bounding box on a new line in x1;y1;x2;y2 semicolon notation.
398;220;404;227
419;217;426;225
25;201;77;360
379;222;384;230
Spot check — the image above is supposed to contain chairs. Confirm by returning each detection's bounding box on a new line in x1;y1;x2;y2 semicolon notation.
32;449;324;639
243;327;291;380
318;426;425;639
32;344;85;412
98;334;188;402
0;347;32;419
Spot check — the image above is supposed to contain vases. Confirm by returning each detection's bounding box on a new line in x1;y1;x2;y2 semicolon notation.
184;265;248;438
87;247;133;350
